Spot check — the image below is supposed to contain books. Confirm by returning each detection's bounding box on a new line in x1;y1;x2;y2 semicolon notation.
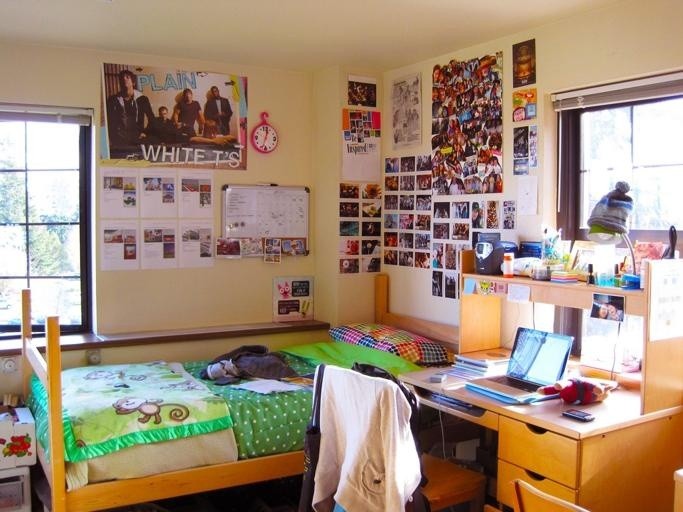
437;348;512;382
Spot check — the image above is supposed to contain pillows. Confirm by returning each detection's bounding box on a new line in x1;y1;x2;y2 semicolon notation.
328;323;454;366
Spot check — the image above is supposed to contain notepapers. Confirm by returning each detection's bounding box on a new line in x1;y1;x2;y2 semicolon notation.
551;271;578;283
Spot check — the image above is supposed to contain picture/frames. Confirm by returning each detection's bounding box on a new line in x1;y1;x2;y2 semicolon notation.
565;241;600;273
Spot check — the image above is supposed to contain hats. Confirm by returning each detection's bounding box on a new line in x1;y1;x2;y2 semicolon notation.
587;191;634;230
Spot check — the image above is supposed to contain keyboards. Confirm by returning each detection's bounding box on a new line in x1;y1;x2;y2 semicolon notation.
431;393;473;411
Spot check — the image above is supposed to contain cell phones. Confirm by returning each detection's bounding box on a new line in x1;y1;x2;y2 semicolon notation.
562;408;595;423
429;373;443;382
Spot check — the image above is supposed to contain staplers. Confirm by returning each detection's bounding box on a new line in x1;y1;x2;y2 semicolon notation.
621;274;640;290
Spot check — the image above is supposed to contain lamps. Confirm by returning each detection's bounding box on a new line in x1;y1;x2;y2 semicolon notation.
587;226;640;290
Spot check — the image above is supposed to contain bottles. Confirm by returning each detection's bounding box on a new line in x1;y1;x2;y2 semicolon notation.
613;262;626;288
586;264;595;287
503;252;514;278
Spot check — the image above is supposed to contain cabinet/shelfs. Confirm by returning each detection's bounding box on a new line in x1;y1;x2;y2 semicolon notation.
398;252;683;511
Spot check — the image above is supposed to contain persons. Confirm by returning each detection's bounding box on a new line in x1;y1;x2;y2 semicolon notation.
201;85;234;137
337;39;556;301
149;105;179;146
172;87;205;140
592;300;608;320
106;69;155;149
604;302;623;321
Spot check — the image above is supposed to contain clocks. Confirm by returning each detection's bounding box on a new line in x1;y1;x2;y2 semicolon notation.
251;111;279;153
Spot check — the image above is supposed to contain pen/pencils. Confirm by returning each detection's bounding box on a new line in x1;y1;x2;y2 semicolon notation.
542;228;562;259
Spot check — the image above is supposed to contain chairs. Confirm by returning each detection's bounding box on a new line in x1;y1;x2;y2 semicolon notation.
507;480;586;512
314;362;485;512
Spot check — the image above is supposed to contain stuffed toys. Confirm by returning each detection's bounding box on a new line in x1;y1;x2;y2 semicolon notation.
535;378;613;405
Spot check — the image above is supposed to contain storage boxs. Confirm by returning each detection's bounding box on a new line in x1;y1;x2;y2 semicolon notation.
0;408;36;469
0;467;29;512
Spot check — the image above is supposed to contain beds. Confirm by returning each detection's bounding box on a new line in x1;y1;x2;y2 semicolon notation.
19;271;501;512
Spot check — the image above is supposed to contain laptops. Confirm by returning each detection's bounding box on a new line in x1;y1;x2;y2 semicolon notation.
464;327;575;404
